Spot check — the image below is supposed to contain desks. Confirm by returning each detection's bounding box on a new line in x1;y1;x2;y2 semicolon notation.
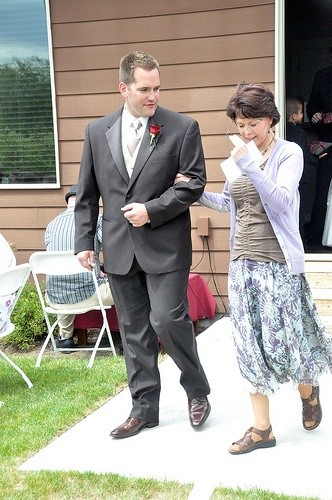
72;271;216;346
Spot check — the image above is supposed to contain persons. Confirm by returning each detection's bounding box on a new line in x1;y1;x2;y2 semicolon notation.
73;51;211;438
44;181;118;355
173;77;332;455
284;97;332;246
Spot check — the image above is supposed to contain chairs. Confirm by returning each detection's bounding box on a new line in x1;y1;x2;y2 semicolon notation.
0;261;34;389
29;250;117;368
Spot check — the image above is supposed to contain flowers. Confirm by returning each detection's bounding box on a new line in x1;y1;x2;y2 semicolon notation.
148;124;161;148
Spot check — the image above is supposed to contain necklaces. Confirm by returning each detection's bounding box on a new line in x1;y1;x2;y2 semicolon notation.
261;135;276;156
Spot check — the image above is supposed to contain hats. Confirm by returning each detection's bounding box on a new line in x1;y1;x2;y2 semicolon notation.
65;184;78;204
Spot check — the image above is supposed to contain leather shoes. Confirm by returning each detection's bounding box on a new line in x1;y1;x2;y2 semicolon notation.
188;394;211;429
109;416;159;438
51;336;74;354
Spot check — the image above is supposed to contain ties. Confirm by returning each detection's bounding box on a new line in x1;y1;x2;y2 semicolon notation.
127;119;143;157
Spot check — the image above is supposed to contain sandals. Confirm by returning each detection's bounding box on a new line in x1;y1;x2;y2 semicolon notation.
228;424;276;455
300;380;322;430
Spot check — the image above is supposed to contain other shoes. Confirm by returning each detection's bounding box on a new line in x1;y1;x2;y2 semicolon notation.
303;244;316;252
